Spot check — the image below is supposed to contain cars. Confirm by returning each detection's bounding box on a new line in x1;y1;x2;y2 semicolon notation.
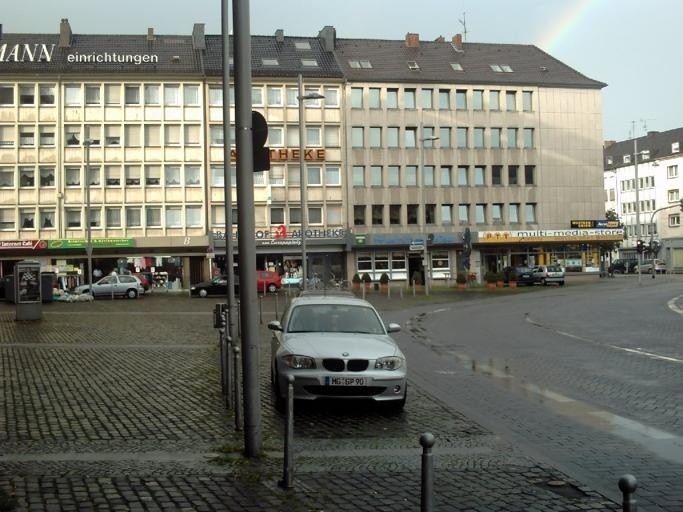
55;269;305;303
482;262;565;290
605;257;667;278
264;293;407;415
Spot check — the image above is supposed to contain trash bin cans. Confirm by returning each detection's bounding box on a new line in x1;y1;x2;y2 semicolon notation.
4;275;15;302
41;274;53;303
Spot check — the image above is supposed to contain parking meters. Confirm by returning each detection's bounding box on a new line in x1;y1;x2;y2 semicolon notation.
210;302;227;400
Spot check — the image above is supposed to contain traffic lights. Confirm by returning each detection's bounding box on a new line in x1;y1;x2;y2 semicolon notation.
679;199;682;212
636;239;643;254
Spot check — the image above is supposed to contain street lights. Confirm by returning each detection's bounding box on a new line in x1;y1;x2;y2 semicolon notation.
82;136;100;304
294;73;327;292
418;122;441;296
632;138;649;282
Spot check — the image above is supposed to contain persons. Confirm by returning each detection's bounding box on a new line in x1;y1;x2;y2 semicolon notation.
21;267;36;282
93;266;103;278
112;267;119;276
